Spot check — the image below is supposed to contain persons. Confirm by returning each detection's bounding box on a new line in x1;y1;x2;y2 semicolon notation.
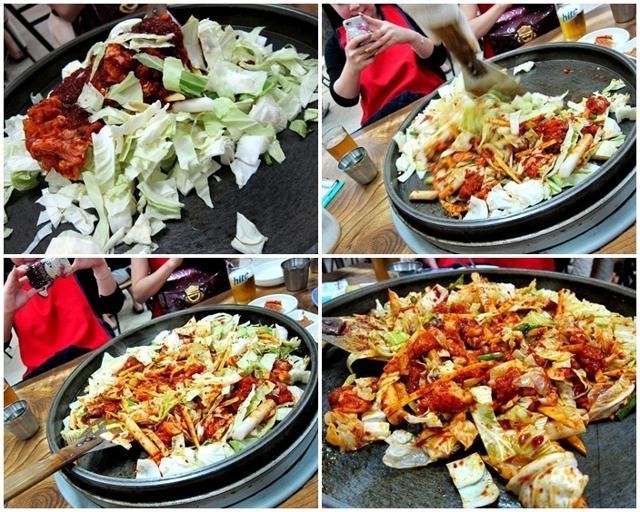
98;267;145;329
322;4;449;133
45;5;177;39
457;4;559;62
4;258;130;378
129;259;230;320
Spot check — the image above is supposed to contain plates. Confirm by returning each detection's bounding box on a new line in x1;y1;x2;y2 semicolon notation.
247;293;299;316
252;259;288;287
387;193;635;253
575;28;631;49
53;431;317;507
286;309;317;343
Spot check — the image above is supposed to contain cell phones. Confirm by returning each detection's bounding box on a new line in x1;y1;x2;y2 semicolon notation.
24;257;72;289
343;14;373;45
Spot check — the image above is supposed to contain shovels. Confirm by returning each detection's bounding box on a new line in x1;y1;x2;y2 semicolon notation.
322;316;392;362
143;4;181;28
3;416;135;503
429;18;526;104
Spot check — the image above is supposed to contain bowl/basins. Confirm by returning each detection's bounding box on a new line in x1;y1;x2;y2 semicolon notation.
5;7;316;258
384;40;637;243
46;303;318;503
323;269;637;507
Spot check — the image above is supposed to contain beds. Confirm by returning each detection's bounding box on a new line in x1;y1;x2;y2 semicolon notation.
321;4;636;255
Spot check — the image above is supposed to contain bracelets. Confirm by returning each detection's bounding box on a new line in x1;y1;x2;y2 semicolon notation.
92;266;112;279
416;36;425;51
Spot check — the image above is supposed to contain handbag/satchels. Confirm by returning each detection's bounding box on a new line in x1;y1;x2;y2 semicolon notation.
157;268;216;314
488;5;560;54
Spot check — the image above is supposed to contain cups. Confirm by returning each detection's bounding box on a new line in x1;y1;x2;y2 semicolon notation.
227;259;258;305
280;258;311;293
337;148;378;189
323;127;360;160
4;400;41;441
553;5;587;43
322;210;342;254
4;377;20;410
